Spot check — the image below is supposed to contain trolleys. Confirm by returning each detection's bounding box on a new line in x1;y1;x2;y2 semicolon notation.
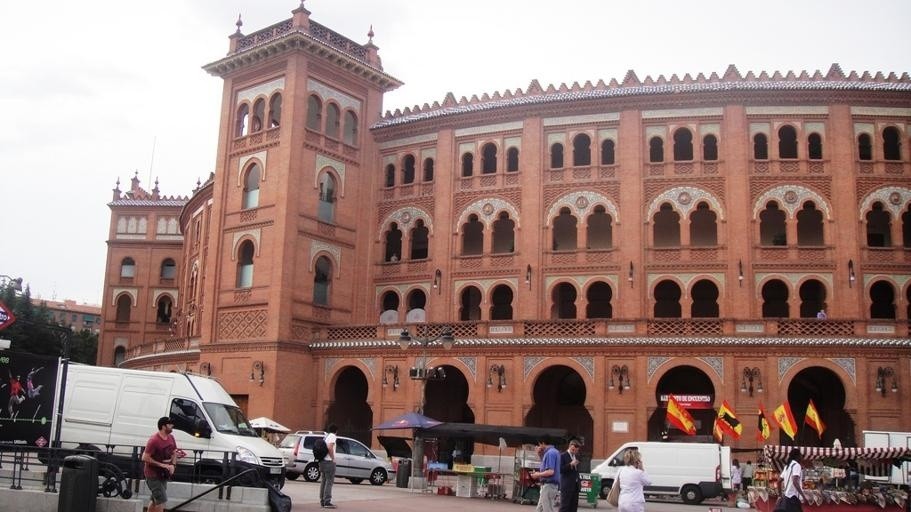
576;471;602;510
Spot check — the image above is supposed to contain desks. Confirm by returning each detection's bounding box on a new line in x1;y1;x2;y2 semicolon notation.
421;469;512;498
748;486;908;512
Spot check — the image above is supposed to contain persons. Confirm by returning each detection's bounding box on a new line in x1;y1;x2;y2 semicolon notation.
611;447;651;512
5;366;24;418
25;365;44;398
740;459;754;496
317;422;337;508
815;306;827;319
256;428;279;446
389;251;397;261
776;448;808;511
729;457;739;492
559;438;583;512
451;444;462;458
141;415;177;511
527;433;562;511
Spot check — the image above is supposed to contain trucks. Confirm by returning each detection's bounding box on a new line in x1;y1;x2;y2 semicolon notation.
42;351;288;494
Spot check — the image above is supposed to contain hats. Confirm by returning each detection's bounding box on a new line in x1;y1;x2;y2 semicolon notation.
158;417;178;425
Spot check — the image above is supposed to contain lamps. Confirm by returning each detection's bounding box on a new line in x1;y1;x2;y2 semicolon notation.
487;364;506;393
848;258;854;288
383;365;399;391
525;265;530;290
199;362;210;376
740;366;763;397
739;259;743;287
628;261;633;287
609;365;630;395
433;269;440;295
876;367;897;397
249;361;264;387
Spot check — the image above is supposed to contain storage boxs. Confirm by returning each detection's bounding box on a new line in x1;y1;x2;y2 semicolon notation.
456;475;477;497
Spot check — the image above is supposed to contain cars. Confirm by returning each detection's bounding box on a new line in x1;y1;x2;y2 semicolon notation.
274;429;396;489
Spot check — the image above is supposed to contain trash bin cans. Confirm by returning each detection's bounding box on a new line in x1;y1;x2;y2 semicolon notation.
58;454;99;512
397;459;411;488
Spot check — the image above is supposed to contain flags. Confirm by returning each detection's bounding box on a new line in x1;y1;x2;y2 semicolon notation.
802;397;825;439
715;400;742;441
664;392;696;436
768;401;798;439
712;416;723;443
755;400;774;443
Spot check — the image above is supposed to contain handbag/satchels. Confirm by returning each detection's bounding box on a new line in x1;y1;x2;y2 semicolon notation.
605;477;620;507
149;453;174;479
772;494;802;512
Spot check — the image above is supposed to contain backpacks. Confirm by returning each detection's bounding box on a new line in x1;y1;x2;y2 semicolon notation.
313;432;332;462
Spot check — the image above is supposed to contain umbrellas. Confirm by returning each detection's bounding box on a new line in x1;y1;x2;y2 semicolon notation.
236;415;290;438
368;411;443;493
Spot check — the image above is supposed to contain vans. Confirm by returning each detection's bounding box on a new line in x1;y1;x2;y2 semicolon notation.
588;441;736;505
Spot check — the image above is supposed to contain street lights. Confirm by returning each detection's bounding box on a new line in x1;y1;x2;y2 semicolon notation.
392;322;454;495
0;272;24;298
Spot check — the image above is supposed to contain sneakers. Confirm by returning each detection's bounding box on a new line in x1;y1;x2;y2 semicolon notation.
321;503;337;508
